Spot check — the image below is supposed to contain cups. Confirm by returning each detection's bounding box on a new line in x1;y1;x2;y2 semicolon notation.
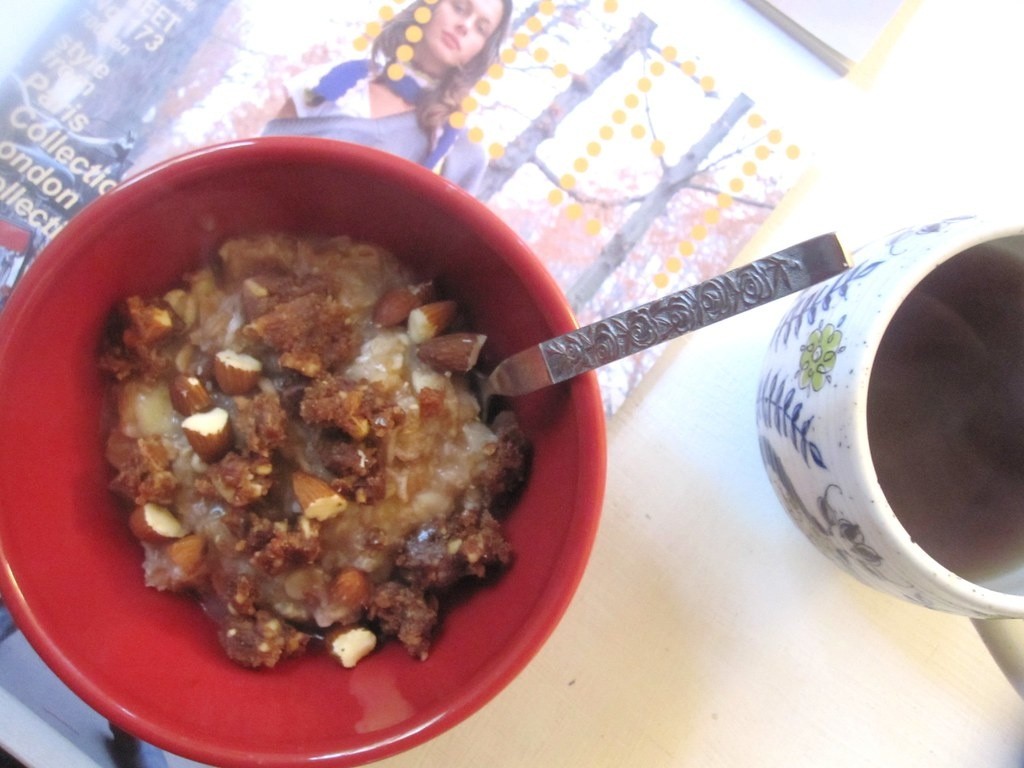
755;213;1022;619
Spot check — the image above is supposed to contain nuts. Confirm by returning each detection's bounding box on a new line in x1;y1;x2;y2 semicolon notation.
117;279;491;667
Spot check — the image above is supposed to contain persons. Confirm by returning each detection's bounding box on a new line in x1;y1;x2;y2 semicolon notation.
0;1;515;768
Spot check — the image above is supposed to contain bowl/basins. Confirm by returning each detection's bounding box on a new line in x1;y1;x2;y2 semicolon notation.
0;139;607;766
